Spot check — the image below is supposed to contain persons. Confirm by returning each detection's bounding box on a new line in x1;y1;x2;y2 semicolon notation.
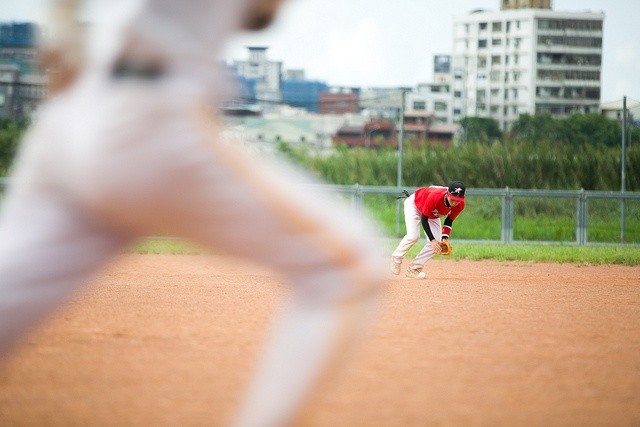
388;180;466;280
1;0;413;427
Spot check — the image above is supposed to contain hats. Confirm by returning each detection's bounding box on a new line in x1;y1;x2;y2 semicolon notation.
447;183;465;201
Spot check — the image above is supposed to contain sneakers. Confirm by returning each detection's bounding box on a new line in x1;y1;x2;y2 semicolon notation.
391;256;402;275
406;265;426;279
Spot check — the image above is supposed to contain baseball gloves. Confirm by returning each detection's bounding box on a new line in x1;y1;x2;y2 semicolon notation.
432;242;452;256
